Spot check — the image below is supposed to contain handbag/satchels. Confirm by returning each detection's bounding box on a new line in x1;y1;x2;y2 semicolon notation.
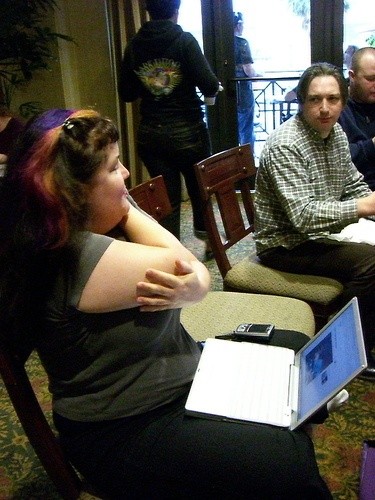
359;439;375;500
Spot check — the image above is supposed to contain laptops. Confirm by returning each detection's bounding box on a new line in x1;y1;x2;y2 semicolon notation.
185;296;368;432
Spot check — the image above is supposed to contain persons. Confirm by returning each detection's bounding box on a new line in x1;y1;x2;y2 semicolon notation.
232;12;263;157
118;0;219;241
343;44;359;78
253;62;375;380
0;91;38;367
0;107;333;500
285;86;298;102
337;47;375;191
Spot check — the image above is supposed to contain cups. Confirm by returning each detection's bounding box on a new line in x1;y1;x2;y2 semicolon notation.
204;81;221;105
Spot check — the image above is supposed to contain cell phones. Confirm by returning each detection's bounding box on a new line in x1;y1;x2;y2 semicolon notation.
234;323;275;337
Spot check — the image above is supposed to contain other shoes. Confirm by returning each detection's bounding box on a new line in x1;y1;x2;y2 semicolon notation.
205;235;227;260
327;387;350;413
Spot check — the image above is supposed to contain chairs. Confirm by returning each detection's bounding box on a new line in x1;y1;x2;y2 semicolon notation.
0;174;316;500
195;143;348;333
